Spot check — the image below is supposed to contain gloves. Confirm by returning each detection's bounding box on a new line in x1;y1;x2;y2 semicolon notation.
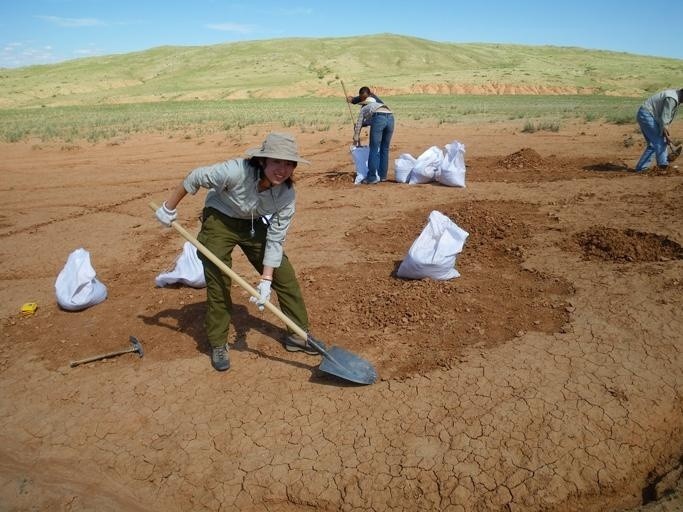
249;279;272;312
156;201;177;227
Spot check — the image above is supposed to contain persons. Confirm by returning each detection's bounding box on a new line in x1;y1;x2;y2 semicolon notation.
633;88;683;172
346;87;387;130
350;97;394;183
154;132;324;371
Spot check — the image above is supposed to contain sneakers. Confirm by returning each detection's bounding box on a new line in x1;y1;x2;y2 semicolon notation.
362;179;387;184
212;344;230;370
286;334;326;355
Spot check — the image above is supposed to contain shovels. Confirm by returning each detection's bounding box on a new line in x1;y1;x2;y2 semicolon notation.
148;202;379;388
341;81;366;148
659;122;682;162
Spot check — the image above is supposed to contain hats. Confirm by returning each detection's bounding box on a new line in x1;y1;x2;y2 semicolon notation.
359;97;376;105
245;134;311;165
